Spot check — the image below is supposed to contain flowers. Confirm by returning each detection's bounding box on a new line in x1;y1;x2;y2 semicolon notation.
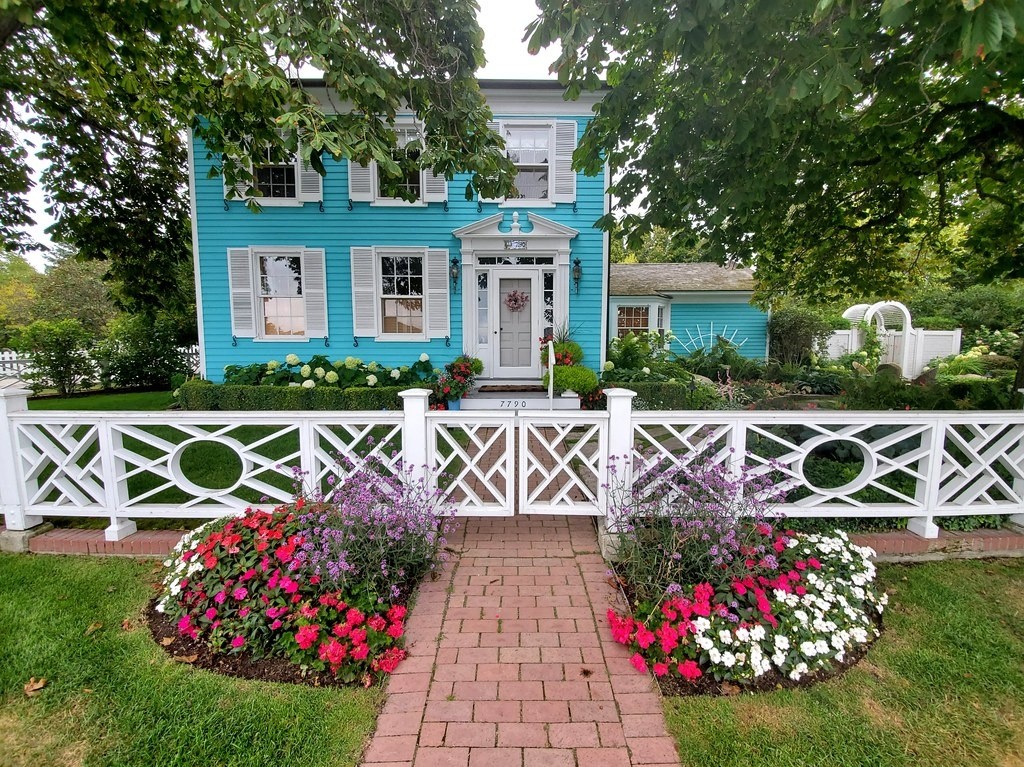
505;289;528;311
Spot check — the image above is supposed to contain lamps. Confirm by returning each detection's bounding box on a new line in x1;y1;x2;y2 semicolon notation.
450;256;460;291
573;257;582;294
